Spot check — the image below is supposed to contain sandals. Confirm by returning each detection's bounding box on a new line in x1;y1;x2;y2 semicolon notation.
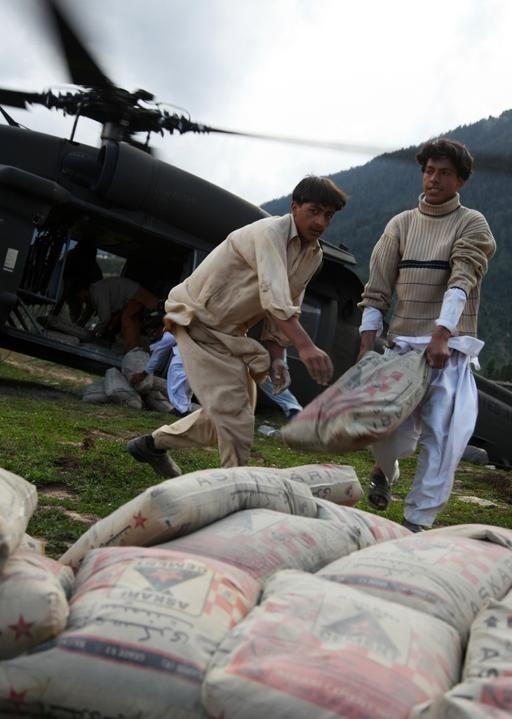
368;463;392;511
402;517;424;534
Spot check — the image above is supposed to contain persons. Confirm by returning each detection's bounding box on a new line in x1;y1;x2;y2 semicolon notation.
127;174;351;484
47;236;105;328
128;314;303;418
355;137;499;533
70;271;158;357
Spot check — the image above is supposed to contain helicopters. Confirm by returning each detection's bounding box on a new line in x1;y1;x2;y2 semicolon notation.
0;0;511;470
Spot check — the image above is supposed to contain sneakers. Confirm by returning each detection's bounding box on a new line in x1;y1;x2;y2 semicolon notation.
126;433;182;479
